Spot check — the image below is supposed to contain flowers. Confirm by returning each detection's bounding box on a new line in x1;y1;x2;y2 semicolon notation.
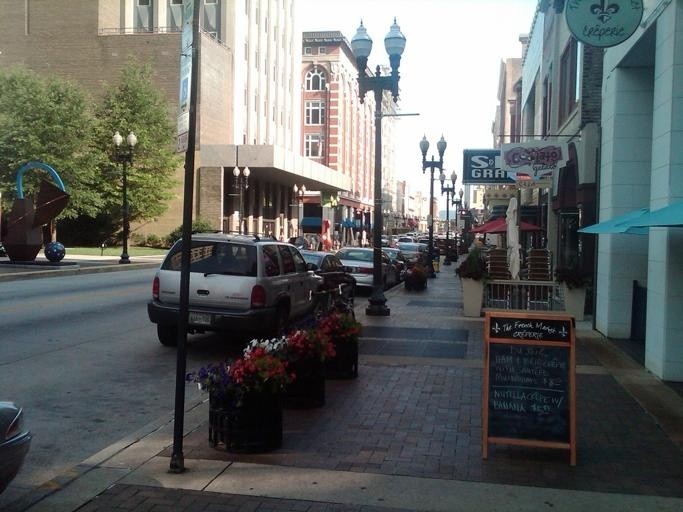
186;303;364;394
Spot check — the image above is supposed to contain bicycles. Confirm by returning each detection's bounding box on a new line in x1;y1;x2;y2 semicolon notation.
302;282;358;331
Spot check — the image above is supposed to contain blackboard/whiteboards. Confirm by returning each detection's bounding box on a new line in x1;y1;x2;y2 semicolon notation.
482;311;576;450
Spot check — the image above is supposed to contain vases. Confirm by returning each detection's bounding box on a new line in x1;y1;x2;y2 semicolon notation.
284;368;325;409
333;336;359;380
206;384;282;454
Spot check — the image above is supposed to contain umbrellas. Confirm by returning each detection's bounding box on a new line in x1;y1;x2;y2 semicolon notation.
575;206;649;235
486;218;544;234
613;201;683;228
467;216;505;248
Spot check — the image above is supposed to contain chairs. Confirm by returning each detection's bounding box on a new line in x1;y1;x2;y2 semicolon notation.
481;247;553;311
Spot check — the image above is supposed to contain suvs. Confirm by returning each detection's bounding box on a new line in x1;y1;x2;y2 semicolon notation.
147;228;327;349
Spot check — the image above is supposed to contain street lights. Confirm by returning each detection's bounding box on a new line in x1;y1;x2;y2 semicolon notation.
355;207;363;247
231;165;252;236
290;182;308;237
382;208;391;225
351;16;409;317
112;128;141;266
413;132;472;282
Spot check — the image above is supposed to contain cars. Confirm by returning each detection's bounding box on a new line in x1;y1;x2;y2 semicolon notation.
0;375;35;502
290;226;459;310
